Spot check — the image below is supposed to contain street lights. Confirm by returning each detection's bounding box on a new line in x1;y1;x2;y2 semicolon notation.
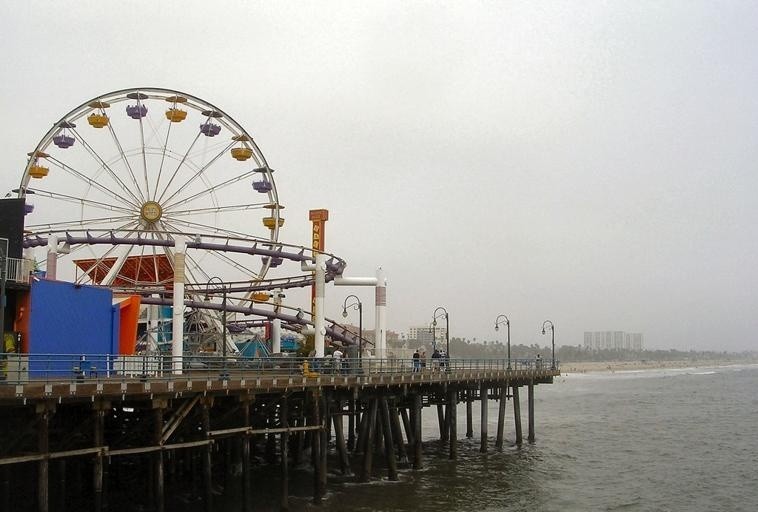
432;307;449;358
428;322;435;353
542;320;555;369
203;276;229;376
342;295;364;374
495;314;511;370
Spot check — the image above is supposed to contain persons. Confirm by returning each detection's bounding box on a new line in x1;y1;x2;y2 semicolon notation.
535;354;543;369
420;351;427;373
413;349;420;372
323;346;350;376
431;349;447;375
